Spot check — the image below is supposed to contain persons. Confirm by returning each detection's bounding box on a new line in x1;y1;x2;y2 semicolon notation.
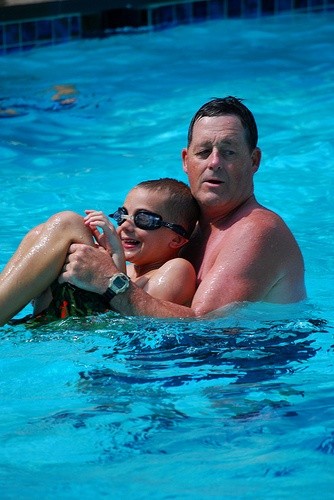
59;96;310;321
0;177;199;328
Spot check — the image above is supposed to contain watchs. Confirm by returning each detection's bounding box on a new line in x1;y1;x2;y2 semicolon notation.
101;272;132;305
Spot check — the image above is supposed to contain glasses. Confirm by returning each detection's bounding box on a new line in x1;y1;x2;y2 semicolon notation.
109;206;192;243
